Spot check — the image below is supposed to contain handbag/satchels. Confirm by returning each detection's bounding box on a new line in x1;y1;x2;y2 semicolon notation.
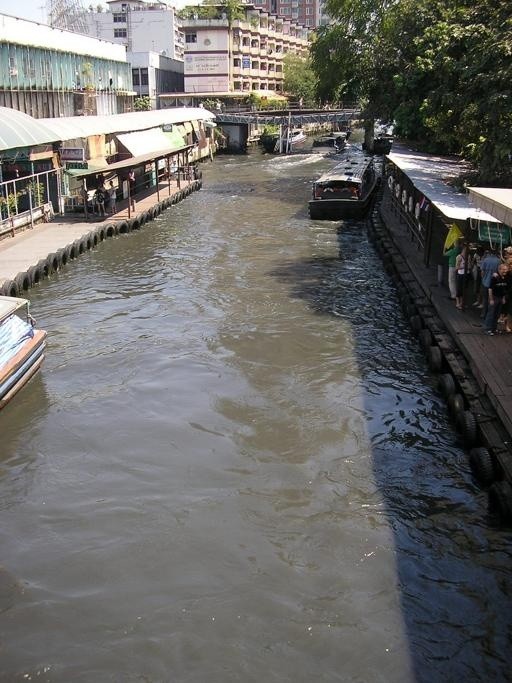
97;194;104;203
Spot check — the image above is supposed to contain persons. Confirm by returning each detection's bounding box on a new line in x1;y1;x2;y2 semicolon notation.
80;180;118;217
443;221;511;335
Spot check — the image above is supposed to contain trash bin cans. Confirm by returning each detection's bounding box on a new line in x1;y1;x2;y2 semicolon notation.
382;137;394;154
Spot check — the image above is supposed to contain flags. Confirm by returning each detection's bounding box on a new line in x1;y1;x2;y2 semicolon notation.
419;194;431;211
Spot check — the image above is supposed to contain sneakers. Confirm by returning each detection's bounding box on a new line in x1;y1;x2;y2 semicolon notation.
455;301;494;336
99;212;109;216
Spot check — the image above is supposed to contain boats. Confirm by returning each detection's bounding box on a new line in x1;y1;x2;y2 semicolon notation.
304;155;380;222
0;294;49;412
277;128;394;156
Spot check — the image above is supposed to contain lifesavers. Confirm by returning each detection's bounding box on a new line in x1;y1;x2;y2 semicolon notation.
341;187;350;192
324;187;333;192
